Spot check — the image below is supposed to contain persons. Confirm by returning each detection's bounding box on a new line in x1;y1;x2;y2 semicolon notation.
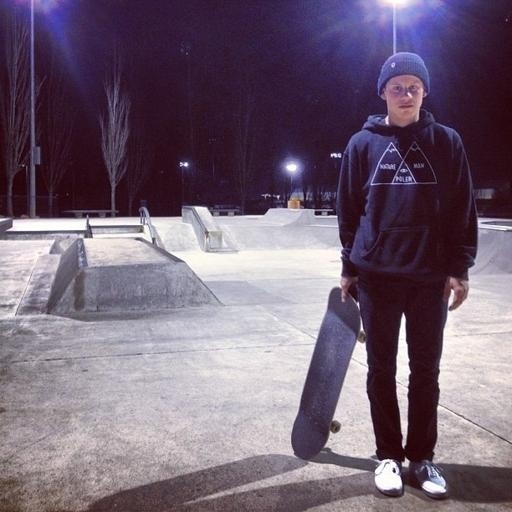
335;52;479;500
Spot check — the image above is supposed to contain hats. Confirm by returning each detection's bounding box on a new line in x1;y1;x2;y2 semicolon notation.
377;51;431;97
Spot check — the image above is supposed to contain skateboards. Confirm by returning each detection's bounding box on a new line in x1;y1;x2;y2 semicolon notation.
291;287;365;460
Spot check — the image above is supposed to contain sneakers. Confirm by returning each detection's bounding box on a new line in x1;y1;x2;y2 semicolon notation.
373;459;403;498
408;459;448;499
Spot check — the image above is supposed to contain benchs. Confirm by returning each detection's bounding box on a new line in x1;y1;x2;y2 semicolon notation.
210;209;240;216
314;208;334;215
65;210;120;217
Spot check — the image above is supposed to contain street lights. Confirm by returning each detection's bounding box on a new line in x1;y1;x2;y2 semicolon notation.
180;161;189;202
287;164;297;193
385;0;404;55
